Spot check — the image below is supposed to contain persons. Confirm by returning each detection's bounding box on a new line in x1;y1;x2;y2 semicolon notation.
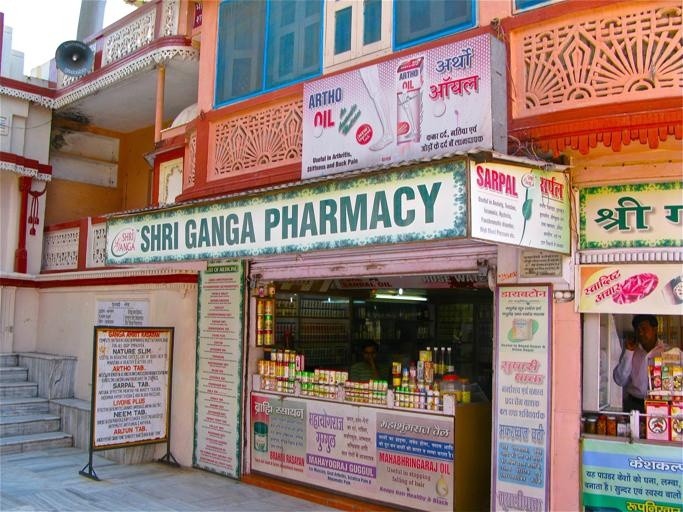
349;339;389;383
612;315;671;423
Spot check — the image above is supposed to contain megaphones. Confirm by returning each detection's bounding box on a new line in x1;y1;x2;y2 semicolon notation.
54;39;94;76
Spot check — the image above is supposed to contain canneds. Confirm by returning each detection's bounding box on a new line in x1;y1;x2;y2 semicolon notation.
257;348;440;413
255;283;276;345
581;415;631;439
